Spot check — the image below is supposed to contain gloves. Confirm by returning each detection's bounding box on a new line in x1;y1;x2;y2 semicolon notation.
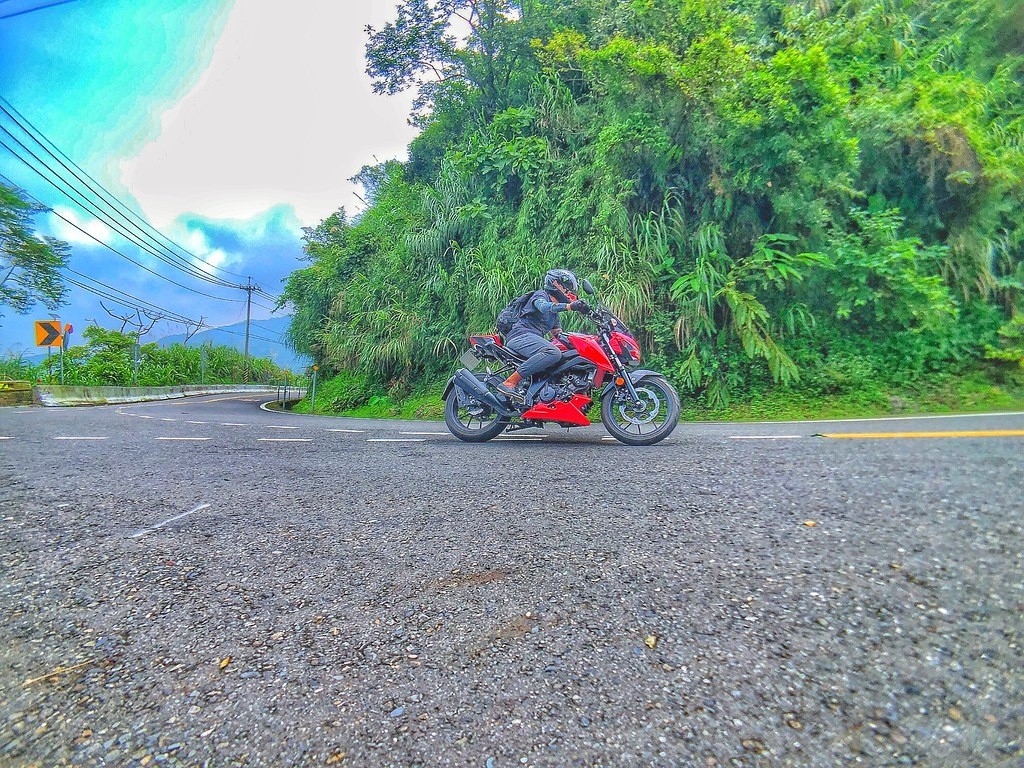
569;298;590;314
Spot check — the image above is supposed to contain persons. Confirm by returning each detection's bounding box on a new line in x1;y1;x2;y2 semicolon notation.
496;269;591;402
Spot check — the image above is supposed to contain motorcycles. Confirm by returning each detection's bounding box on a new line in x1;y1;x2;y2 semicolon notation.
440;278;681;446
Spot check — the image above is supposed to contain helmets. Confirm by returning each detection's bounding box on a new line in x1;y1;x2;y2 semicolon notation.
544;269;580;303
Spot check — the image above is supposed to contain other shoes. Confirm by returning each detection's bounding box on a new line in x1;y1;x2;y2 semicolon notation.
496;382;524;403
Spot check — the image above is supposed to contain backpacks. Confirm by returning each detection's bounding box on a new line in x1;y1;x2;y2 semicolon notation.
496;291;549;335
